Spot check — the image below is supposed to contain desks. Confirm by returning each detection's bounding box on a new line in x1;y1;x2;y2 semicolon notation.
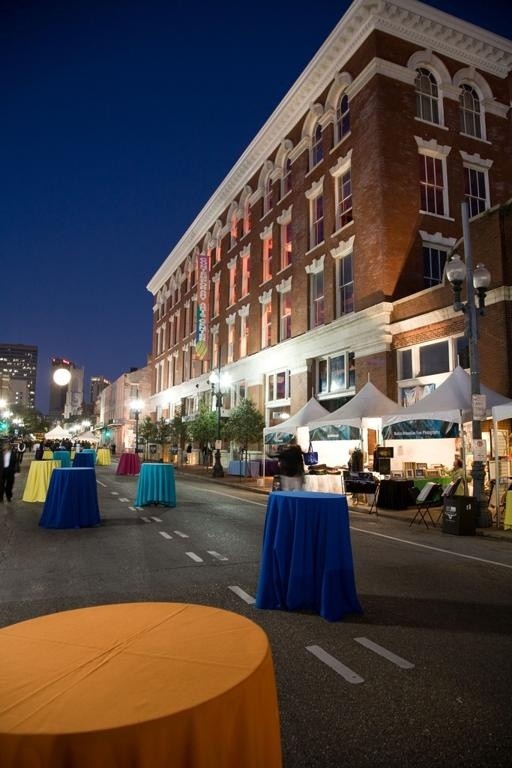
344;480;378;505
254;490;366;623
0;602;284;768
228;459;280;477
377;477;452;509
21;447;176;531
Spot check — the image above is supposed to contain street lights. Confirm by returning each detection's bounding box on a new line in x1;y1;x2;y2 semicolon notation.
209;370;230;476
131;399;144;456
445;253;493;527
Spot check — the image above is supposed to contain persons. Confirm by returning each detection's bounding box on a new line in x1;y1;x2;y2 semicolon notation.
0;440;21;502
277;438;306;492
452;454;463;472
17;440;26;463
27;437;98;455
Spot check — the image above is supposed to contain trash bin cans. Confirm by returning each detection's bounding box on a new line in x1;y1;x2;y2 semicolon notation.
443;497;476;535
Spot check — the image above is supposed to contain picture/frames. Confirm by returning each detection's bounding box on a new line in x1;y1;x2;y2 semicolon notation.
375;447;439;479
